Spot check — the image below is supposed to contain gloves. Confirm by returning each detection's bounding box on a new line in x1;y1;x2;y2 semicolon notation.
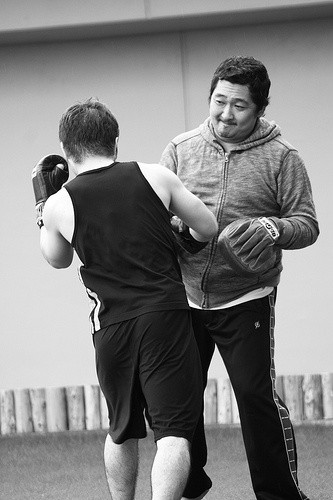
171;215;209;256
217;216;284;279
31;153;70;228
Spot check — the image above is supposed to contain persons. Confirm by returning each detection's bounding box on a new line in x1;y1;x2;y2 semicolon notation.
31;100;218;499
159;56;322;500
